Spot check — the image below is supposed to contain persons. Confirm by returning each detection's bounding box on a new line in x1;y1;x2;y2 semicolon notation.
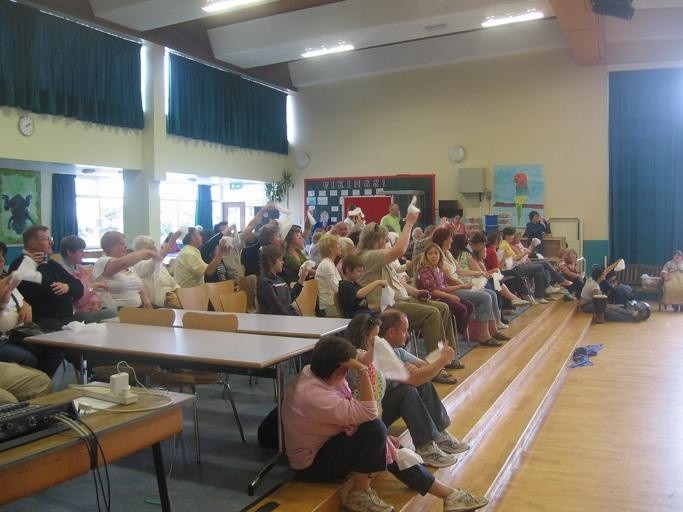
281;334;490;512
1;361;52;407
0;241;65;379
578;257;642;322
660;249;683;313
343;312;386;425
371;307;472;470
8;224;110;385
59;194;587;384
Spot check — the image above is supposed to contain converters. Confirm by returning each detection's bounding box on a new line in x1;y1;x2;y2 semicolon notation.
109;372;131;397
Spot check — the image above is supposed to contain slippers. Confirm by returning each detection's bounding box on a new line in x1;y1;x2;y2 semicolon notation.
480;317;510;346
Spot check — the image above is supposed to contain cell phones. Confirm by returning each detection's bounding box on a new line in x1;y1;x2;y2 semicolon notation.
410;196;417;207
416;293;431;298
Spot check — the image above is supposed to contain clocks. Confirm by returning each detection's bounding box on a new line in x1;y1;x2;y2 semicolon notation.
18;115;36;136
447;145;465;163
295;153;310;167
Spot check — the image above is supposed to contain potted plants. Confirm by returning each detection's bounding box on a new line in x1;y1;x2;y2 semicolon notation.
262;169;295;218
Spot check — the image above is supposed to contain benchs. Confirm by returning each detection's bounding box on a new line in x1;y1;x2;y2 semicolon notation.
601;264;683;312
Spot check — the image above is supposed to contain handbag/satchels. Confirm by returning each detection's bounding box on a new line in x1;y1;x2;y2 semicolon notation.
6;324;50;355
51;355;82;410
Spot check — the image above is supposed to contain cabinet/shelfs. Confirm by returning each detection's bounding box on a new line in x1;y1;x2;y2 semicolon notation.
519;236;566;268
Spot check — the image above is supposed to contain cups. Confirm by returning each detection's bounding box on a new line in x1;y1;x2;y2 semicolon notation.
40;254;48;264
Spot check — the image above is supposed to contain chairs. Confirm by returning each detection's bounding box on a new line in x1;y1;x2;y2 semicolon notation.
91;307;175;385
174;271;270;403
289;281;320;317
151;310;246;464
332;294;344;318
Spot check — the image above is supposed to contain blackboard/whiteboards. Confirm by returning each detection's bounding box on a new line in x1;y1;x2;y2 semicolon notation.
303;174;436;244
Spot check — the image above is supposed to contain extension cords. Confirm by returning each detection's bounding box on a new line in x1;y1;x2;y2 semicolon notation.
82;386;139;406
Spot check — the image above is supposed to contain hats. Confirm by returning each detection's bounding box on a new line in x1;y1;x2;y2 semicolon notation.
348;205;361;216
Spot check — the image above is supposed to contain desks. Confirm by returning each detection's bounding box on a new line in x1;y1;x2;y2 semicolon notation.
23;323;320;495
0;380;195;511
100;308;353;404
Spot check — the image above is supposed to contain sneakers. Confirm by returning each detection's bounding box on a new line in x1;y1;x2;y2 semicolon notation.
511;280;573;306
344;487;394;512
435;431;470;454
443;488;489;512
415;440;458;467
432;369;457;384
445;360;464;369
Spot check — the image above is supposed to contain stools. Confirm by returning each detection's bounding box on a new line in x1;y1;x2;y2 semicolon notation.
404;325;425;359
448;308;471;354
